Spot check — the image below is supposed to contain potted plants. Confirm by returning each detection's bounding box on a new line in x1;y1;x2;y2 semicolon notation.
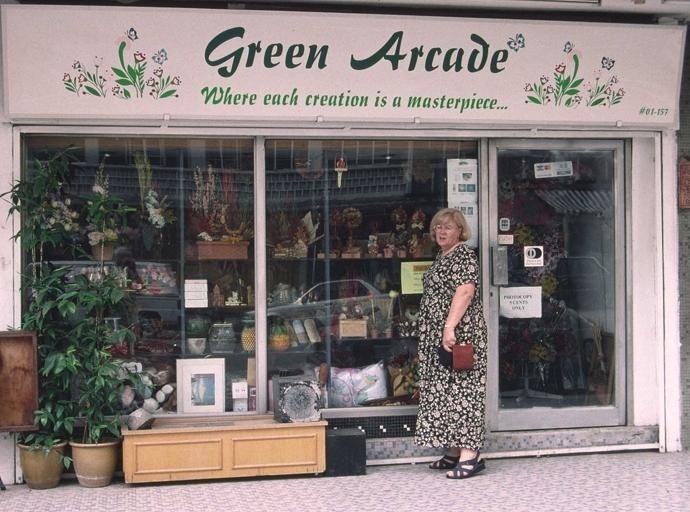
10;265;83;490
68;275;155;487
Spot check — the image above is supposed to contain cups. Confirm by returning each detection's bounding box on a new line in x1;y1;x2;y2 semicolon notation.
189;338;206;356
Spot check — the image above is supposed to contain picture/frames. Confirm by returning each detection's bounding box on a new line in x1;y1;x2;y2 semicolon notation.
183;366;221;414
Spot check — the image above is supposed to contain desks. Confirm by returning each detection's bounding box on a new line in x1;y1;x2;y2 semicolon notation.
120;415;329;487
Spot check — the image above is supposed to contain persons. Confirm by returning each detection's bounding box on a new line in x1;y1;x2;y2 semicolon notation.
407;205;492;482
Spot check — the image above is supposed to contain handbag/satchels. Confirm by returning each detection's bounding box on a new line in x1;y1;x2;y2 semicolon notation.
451;343;474;370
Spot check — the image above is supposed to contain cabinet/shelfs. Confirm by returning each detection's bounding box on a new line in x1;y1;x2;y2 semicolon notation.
30;260;181;364
185;257;436;358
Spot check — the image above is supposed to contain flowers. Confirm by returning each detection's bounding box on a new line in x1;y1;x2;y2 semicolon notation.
78;184;137;247
137;181;178;228
29;182;82;248
185;165;246;241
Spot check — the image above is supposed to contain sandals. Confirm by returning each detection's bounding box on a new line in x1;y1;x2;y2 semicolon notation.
429;454;460;469
447;449;486;479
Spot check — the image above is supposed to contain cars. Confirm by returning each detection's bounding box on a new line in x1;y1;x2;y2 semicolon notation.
240;278;395;329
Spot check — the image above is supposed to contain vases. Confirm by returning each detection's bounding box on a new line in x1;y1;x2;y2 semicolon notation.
185;240;250;260
142;223;173;259
39;238;73;260
92;244;113;261
